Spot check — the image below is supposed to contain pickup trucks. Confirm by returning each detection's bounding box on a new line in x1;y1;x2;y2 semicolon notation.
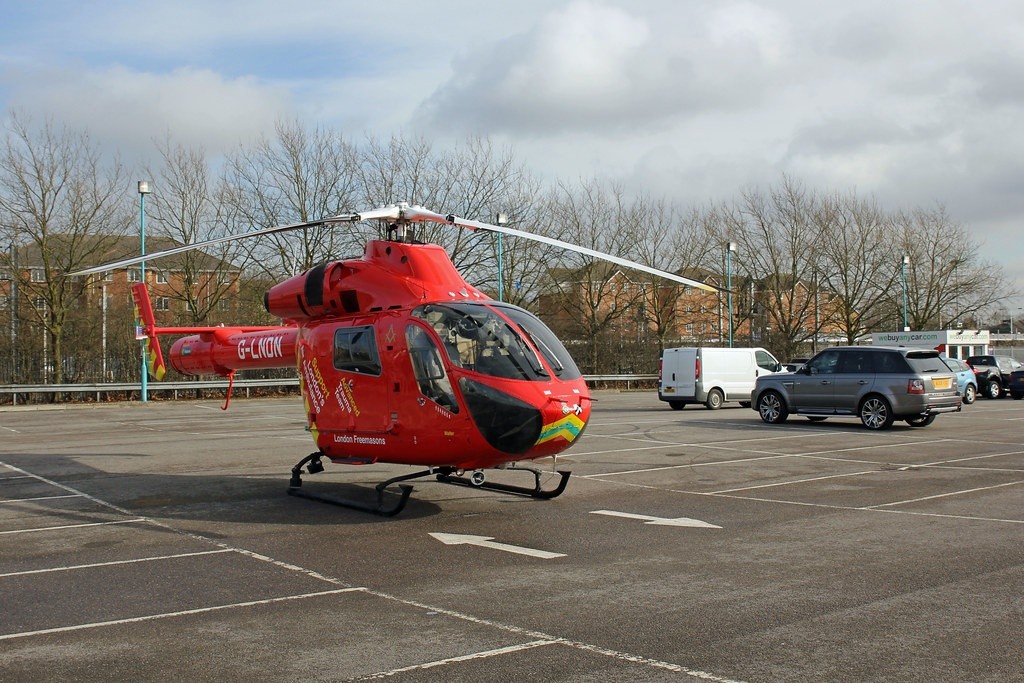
961;354;1022;400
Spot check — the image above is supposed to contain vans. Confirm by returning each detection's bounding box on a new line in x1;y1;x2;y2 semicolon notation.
657;346;790;410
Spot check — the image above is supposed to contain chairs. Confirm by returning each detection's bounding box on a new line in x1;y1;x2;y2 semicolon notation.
414;322;466;409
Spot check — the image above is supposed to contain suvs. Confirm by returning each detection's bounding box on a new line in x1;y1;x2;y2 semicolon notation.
750;345;962;430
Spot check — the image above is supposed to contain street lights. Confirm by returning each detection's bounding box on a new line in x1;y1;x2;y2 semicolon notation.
901;254;912;332
495;213;509;302
996;307;1022;357
726;242;738;347
137;179;155;399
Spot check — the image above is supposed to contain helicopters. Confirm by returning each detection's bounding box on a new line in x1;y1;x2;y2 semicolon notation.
64;198;720;516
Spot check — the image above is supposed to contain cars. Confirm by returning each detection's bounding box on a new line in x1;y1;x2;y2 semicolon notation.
939;358;979;405
1008;364;1024;401
781;364;807;375
